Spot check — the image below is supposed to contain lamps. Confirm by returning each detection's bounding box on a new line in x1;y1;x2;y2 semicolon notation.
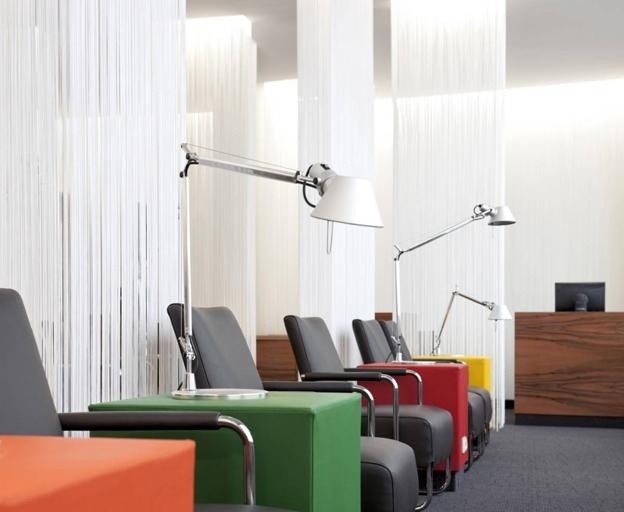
392;203;516;365
170;141;385;401
428;284;511;356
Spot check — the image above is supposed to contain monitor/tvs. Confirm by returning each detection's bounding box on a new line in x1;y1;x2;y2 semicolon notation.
555;282;605;312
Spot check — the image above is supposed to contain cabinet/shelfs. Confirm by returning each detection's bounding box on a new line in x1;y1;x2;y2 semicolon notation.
515;312;624;427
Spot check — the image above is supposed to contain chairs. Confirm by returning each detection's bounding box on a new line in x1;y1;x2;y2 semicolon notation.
166;303;421;510
380;319;493;447
1;287;271;510
282;313;454;509
351;318;485;472
